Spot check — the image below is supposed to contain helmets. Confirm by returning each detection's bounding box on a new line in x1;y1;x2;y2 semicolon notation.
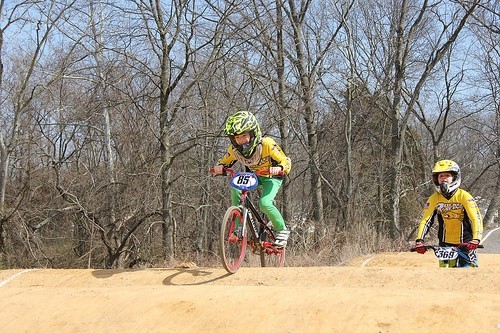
225;110;262;158
431;160;460;193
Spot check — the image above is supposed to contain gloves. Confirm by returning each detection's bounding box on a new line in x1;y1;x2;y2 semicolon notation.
416;239;427;254
467;240;479;250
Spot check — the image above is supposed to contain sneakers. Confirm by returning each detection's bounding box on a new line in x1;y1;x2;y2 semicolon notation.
271;225;291;247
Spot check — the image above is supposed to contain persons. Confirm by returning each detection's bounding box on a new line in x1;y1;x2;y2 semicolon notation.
214;111;291;249
415;159;483;268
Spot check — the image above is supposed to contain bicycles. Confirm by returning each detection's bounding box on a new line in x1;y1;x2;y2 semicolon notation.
410;243;484;267
209;167;285;274
